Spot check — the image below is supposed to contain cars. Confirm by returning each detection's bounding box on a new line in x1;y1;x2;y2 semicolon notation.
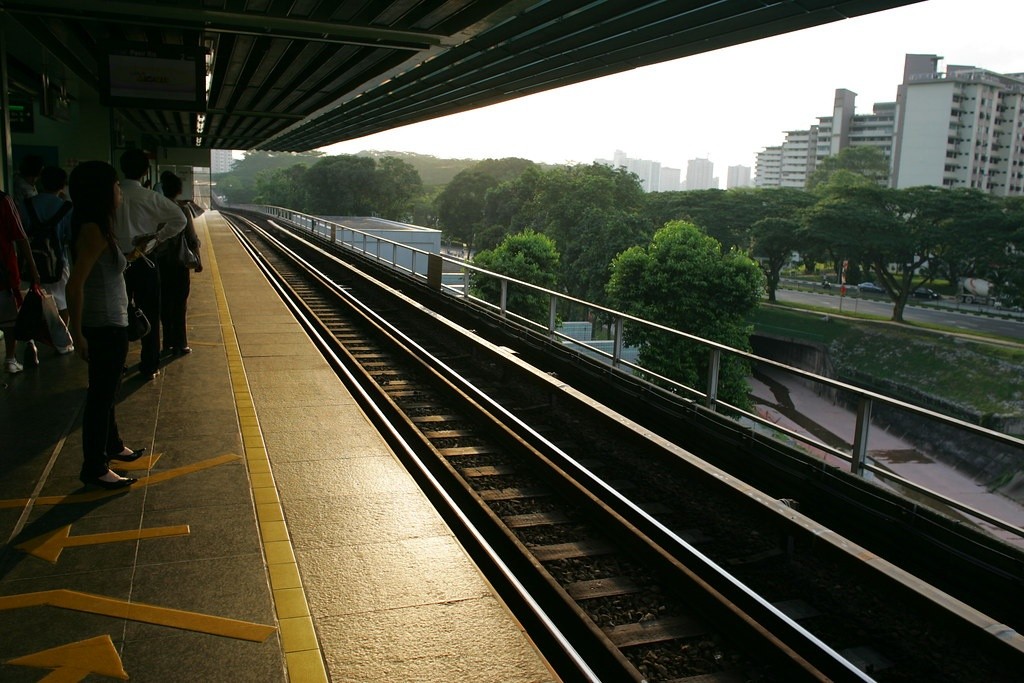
913;287;941;299
857;282;885;293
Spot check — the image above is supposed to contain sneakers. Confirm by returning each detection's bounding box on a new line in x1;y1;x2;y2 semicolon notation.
3;357;23;373
24;339;39;366
58;345;75;354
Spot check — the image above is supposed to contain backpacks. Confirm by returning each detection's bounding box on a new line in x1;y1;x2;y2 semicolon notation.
17;196;72;282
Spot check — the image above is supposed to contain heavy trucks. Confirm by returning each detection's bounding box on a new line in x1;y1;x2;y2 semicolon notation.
955;275;1006;307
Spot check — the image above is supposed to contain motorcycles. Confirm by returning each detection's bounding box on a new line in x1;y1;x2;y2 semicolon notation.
823;280;831;288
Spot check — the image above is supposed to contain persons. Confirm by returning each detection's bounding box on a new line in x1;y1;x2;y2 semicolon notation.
0;148;204;489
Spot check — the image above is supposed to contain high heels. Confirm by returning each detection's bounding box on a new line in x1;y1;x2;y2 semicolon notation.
106;447;146;464
80;469;138;489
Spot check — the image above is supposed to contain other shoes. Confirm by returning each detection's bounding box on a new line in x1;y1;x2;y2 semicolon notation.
145;368;160;380
164;343;193;352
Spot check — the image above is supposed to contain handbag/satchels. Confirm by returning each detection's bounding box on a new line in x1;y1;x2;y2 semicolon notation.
127;305;151;342
15;282;73;349
176;230;200;269
